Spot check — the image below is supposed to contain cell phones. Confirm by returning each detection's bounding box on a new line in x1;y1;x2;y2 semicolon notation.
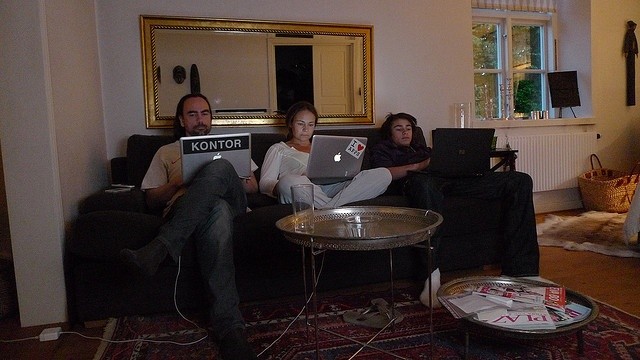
111;183;134;188
105;189;130;195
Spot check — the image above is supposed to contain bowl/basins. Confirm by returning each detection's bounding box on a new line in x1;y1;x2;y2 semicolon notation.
344;214;384;239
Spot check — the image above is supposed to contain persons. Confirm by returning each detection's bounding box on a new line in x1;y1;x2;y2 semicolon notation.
258;100;394;209
367;112;542;310
119;93;261;360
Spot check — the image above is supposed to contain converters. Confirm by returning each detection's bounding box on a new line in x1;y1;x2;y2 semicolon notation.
39;328;62;342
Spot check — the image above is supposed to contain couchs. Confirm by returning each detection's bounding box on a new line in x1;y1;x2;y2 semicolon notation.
62;124;542;329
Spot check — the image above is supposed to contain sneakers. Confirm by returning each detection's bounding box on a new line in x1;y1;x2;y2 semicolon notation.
221;329;256;360
118;239;168;285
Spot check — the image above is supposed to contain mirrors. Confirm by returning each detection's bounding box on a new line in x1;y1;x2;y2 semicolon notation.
138;12;376;131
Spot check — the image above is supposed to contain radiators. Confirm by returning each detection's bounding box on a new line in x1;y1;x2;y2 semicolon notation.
500;129;597;193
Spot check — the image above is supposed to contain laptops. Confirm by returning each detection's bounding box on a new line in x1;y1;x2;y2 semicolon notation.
179;133;251;185
304;135;368;185
407;129;495;177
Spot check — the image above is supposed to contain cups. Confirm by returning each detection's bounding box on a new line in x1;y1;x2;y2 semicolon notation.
291;183;315;234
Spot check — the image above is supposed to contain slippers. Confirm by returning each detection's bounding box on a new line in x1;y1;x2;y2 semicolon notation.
371;298;404;324
342;305;393;327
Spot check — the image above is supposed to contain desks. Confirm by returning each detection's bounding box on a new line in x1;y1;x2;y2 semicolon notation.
275;204;445;360
437;275;600;358
488;148;519;173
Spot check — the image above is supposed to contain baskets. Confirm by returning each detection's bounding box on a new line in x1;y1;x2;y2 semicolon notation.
579;154;640;213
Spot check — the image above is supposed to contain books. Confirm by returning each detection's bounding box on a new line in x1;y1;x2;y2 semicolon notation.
437;287;592;330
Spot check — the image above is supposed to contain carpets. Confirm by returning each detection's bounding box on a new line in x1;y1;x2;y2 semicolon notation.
537;209;640;260
92;275;640;359
